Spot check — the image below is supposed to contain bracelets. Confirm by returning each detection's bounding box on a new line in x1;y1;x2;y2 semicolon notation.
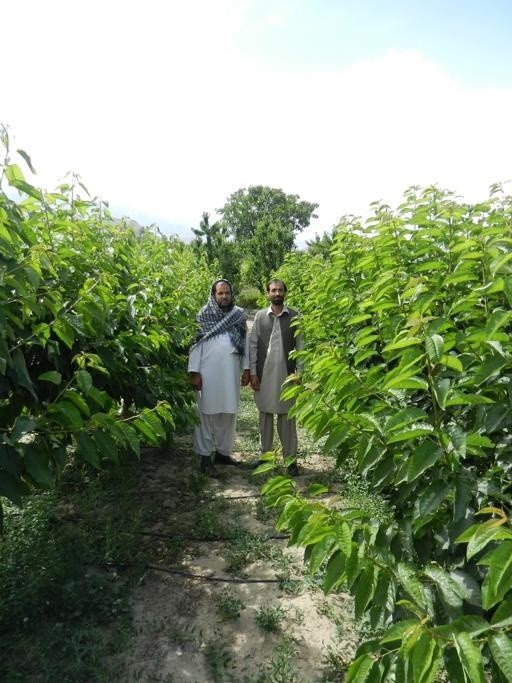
245;370;250;371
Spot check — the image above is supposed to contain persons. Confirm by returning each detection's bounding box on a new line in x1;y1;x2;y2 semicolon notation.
187;278;250;478
250;278;305;476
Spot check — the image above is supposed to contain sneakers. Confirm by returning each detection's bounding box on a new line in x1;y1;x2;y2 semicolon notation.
287;463;300;476
201;456;219;478
217;453;238;466
